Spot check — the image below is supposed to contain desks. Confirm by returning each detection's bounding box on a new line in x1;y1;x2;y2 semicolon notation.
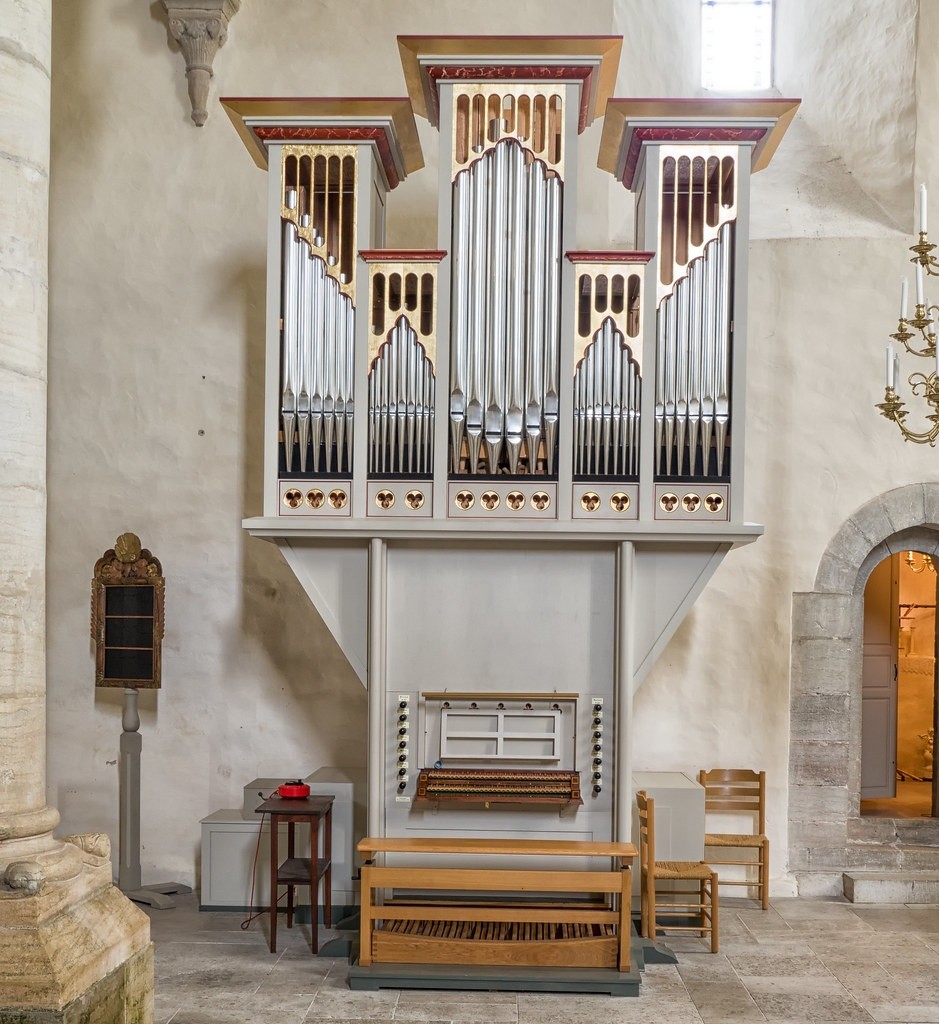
357;837;639;973
255;795;335;955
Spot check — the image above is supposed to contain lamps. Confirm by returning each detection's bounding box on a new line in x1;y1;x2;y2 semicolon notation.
905;552;934;573
873;182;939;446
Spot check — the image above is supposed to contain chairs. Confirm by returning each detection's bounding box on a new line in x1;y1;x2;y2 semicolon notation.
634;789;720;954
699;768;769;911
